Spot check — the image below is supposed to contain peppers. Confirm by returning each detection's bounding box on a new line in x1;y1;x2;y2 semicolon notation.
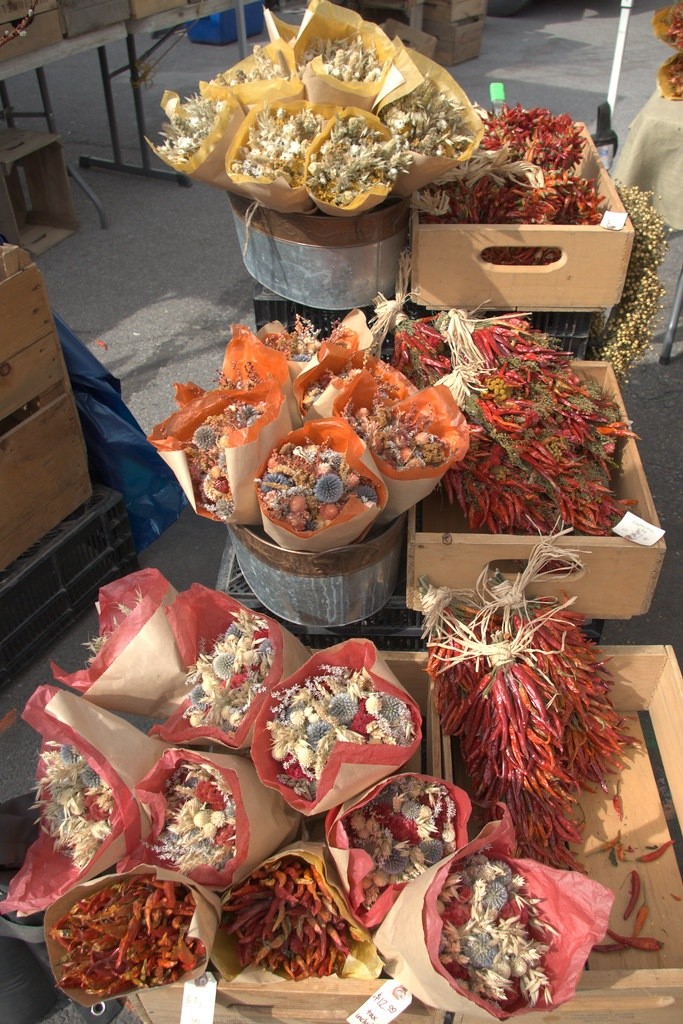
393;314;644;539
219;856;366;981
47;876;206;1000
419;101;608;266
422;588;672;952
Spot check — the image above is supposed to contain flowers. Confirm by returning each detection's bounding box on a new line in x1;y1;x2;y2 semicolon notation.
651;0;683;100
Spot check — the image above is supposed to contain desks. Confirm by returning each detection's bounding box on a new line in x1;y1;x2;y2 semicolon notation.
615;90;683;363
0;21;131;231
78;0;255;187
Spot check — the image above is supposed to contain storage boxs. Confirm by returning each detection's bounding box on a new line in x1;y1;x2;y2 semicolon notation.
252;279;395;364
0;128;80;259
445;642;683;1024
345;0;487;66
0;482;141;691
409;122;635;312
486;312;590;360
183;0;263;44
406;358;667;620
120;649;442;1024
131;0;188;21
0;0;63;66
60;0;131;39
0;261;93;569
